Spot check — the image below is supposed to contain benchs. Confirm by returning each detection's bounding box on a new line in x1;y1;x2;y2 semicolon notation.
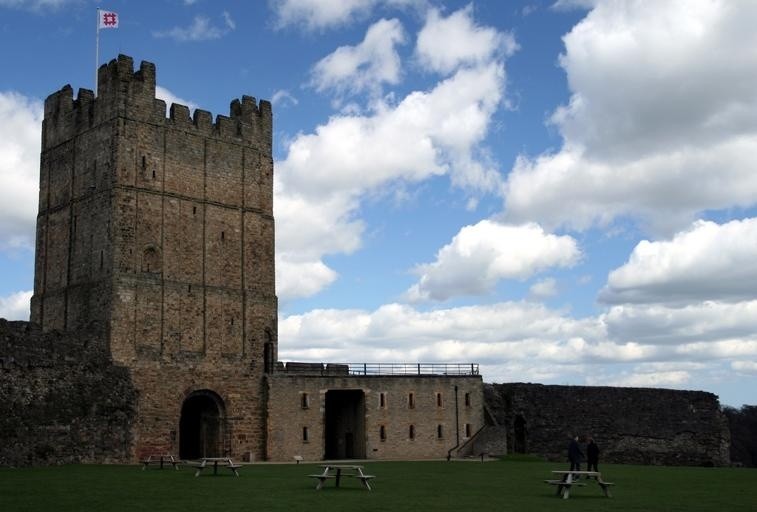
303;463;376;491
191;455;242;477
542;468;615;501
137;453;184;472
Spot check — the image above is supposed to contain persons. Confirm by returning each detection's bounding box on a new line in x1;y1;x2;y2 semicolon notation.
586;438;600;480
568;435;585;480
